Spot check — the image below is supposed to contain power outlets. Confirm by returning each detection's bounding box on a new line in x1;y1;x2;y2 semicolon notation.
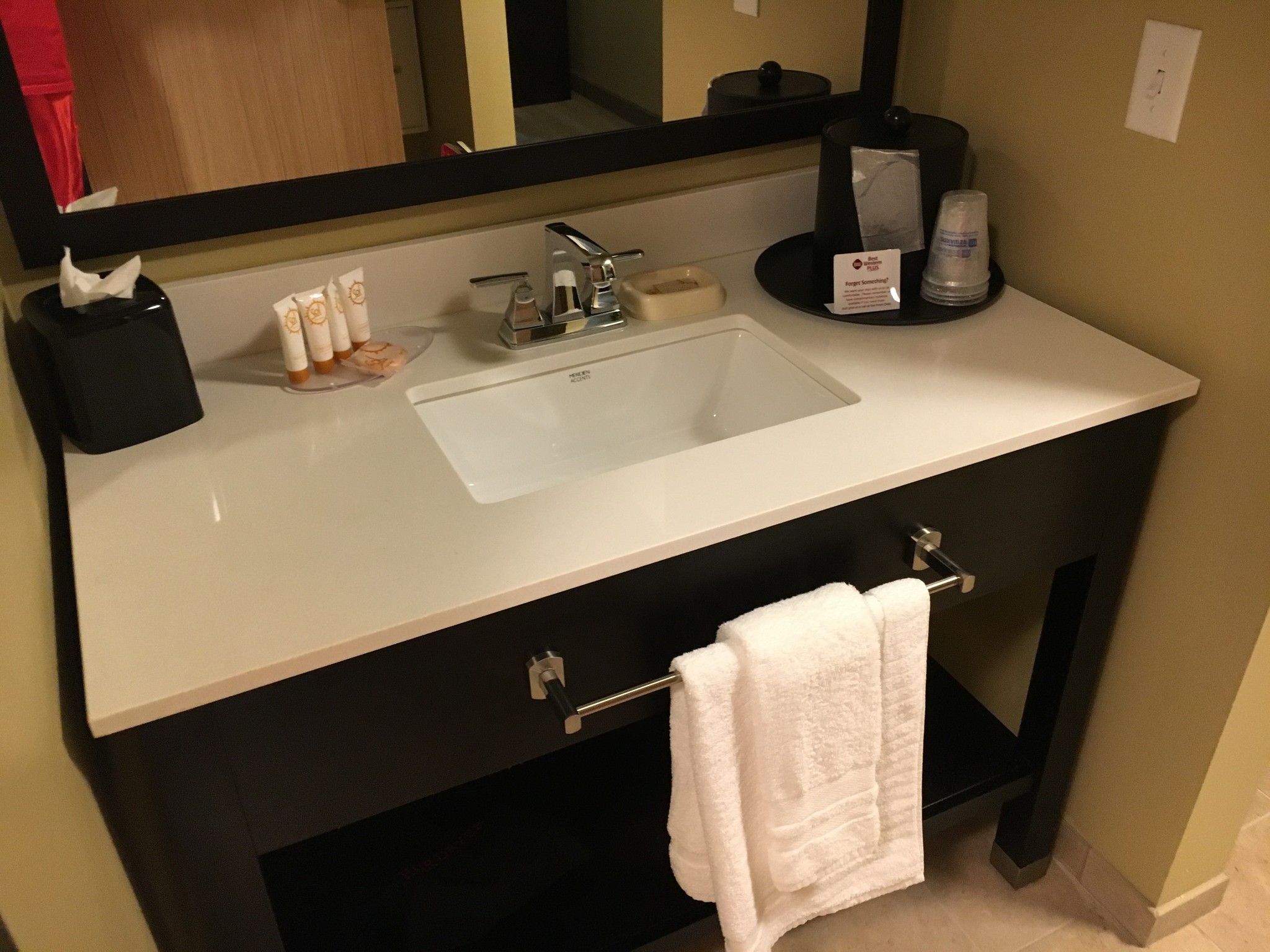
1121;19;1205;146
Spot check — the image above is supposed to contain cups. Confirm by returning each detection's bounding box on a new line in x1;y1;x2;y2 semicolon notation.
919;188;993;308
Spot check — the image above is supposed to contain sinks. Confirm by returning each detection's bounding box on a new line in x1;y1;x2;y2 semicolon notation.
404;310;861;507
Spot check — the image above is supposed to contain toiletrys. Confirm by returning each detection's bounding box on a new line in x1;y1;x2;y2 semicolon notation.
338;266;374;348
325;276;354;360
293;286;338;376
270;293;312;386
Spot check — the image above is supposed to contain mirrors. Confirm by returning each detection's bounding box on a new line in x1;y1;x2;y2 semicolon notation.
0;1;905;272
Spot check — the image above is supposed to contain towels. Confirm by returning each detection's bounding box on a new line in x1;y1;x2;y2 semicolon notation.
715;577;884;893
665;575;930;952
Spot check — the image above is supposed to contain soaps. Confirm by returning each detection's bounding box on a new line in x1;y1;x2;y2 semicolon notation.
354;338;412;384
653;275;701;299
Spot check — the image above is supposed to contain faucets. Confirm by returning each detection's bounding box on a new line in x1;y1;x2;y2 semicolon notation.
543;219;622;340
438;136;474;158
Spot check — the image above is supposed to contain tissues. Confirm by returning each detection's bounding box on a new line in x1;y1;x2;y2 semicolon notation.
63;185;121;213
20;244;205;457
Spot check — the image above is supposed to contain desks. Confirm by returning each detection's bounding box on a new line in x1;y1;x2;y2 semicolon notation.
45;243;1194;952
